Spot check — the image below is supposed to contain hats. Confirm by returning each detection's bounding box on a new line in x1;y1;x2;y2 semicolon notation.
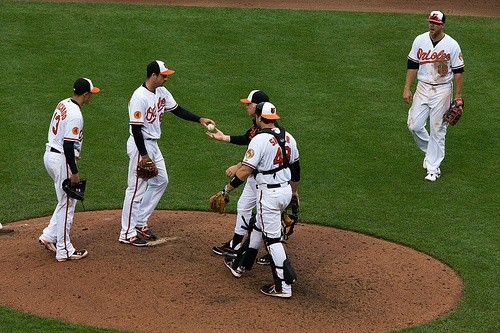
240;90;269;104
147;60;175;75
256;102;280;119
74;78;100;93
428;11;445;24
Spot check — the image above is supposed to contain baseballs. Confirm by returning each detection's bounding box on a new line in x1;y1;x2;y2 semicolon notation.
208;124;215;131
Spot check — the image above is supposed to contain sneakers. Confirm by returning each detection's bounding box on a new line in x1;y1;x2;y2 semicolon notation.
119;233;148;246
39;237;56;253
56;249;88;261
256;254;271;265
260;282;292;297
135;227;157;240
224;256;245;278
212;239;242;256
425;167;441;181
423;158;427;169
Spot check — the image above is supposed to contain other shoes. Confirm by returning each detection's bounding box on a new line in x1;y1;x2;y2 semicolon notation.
0;228;14;235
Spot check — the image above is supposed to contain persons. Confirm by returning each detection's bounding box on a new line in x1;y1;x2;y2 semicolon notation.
209;101;300;298
402;11;465;181
39;78;100;261
118;60;215;246
205;89;278;265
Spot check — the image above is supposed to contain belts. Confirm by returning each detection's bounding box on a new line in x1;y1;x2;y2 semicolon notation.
146;138;156;140
256;181;289;189
50;147;61;153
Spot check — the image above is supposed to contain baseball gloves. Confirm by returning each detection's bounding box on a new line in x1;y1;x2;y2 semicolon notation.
62;178;88;201
136;157;158;181
281;193;299;241
209;191;230;214
442;104;464;126
